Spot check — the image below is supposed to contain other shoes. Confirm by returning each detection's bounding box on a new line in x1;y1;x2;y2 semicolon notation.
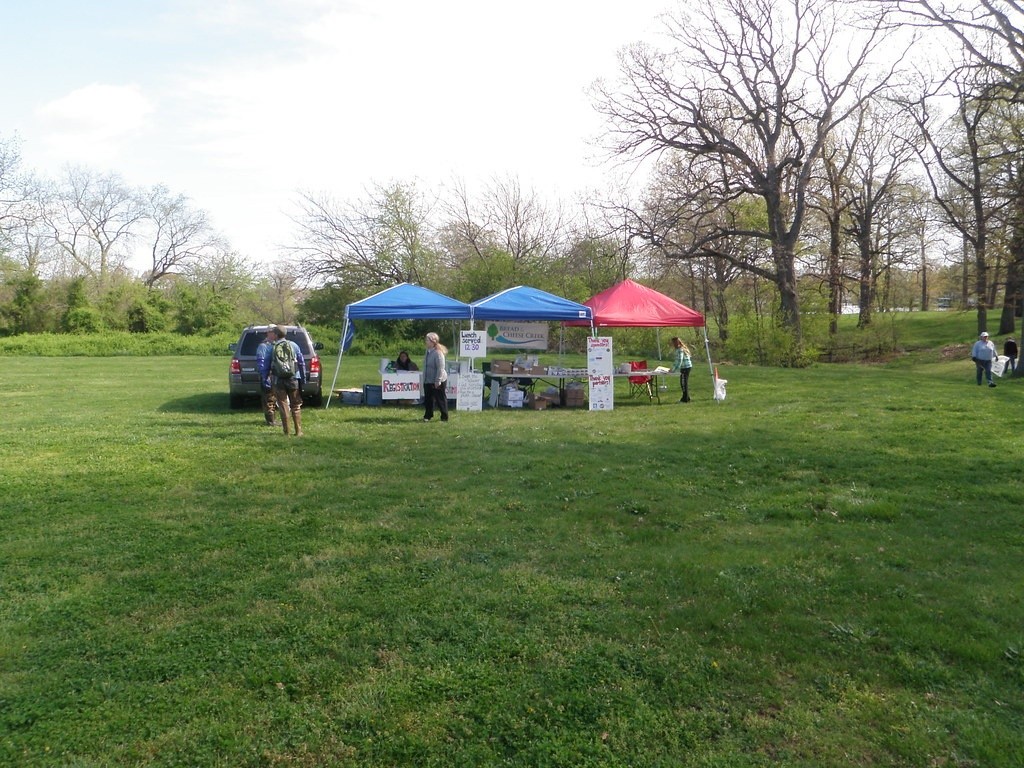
989;383;996;387
268;421;283;427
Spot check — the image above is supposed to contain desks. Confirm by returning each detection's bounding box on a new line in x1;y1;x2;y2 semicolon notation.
485;372;661;409
378;370;458;408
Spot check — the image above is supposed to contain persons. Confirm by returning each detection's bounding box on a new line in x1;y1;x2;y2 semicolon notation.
670;337;691;403
423;333;448;421
256;324;307;438
395;351;419;371
971;332;998;387
1003;336;1018;374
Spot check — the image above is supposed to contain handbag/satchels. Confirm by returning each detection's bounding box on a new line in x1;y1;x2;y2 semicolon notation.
714;368;728;400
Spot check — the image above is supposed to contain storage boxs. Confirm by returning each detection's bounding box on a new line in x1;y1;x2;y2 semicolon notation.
339;392;362;404
491;360;589;410
365;385;382;406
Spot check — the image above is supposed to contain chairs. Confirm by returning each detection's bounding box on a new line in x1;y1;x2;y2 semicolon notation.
482;364;502;390
510;361;535;393
627;360;652;399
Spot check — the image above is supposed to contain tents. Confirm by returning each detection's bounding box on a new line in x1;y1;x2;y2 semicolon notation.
325;281;472;410
557;278;720;404
468;284;594;390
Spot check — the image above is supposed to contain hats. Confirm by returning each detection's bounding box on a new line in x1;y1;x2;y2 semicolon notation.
275;326;287;337
981;332;988;336
267;324;276;333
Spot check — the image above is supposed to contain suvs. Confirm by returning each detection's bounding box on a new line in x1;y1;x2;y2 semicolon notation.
228;322;324;409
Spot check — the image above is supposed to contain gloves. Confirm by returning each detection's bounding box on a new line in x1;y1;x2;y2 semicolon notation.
972;357;976;362
996;356;999;362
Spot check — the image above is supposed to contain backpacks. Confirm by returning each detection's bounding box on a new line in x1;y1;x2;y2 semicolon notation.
271;340;295;378
